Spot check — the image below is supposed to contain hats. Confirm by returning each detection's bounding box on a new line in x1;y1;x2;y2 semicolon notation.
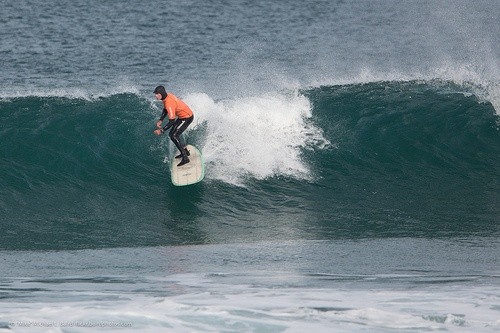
153;86;167;100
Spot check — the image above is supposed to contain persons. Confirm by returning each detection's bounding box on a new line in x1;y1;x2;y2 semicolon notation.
153;84;195;167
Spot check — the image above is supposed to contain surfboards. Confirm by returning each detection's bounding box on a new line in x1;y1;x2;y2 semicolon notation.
171;145;204;187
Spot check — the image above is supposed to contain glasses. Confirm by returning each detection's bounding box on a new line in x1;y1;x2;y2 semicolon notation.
156;92;160;94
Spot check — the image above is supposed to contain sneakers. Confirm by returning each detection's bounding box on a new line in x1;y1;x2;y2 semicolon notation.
175;149;191;159
177;157;190;166
